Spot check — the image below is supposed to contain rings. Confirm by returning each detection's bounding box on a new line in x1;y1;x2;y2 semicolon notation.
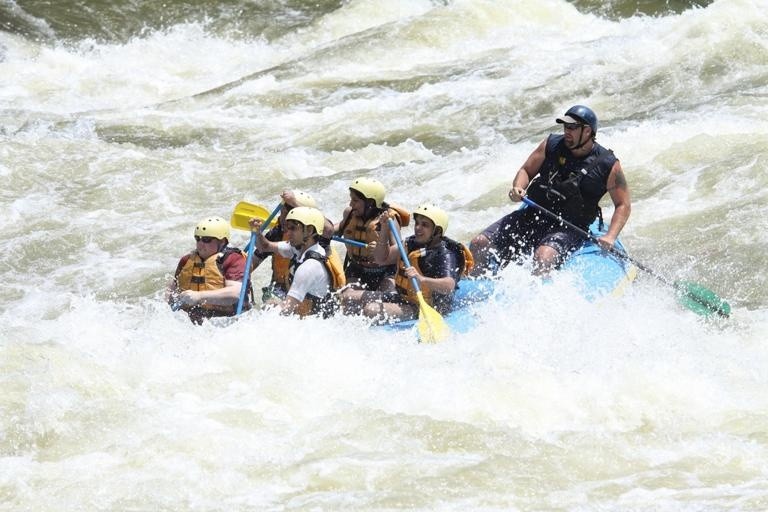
509;193;512;197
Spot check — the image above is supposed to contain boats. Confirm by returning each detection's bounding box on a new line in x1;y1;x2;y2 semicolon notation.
373;201;637;347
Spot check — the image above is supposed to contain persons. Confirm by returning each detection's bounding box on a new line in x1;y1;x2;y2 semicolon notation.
468;105;631;283
245;188;335;302
248;206;337;319
331;176;403;292
167;215;254;325
343;202;466;327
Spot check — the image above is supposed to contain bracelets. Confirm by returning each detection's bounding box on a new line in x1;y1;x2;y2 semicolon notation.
377;241;388;246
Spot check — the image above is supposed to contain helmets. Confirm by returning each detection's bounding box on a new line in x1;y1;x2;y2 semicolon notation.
194;176;448;252
565;105;597;134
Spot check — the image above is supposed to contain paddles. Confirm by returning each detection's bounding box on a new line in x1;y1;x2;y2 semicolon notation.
521;195;730;322
231;202;369;251
388;218;450;341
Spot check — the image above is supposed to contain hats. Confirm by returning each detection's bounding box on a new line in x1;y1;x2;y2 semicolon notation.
557;115;582;125
